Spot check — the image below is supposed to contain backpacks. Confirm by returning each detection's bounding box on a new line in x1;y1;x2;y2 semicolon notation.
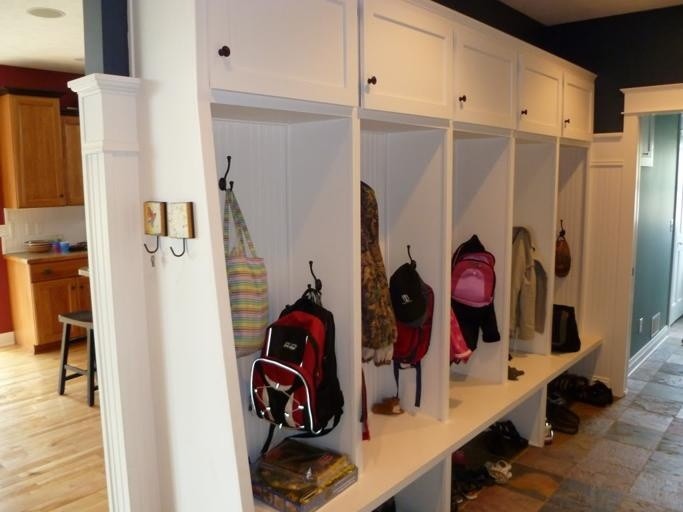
451;252;496;309
248;288;344;435
392;286;433;367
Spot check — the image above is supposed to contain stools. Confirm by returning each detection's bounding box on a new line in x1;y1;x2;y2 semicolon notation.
58;311;97;407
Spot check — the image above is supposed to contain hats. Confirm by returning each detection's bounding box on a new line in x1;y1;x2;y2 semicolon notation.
389;265;426;326
556;239;570;277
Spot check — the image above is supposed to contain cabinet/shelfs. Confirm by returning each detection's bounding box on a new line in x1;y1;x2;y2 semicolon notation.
141;1;605;512
4;254;90;349
0;95;66;211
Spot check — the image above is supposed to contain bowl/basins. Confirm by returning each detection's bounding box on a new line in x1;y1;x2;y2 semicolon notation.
24;239;52;252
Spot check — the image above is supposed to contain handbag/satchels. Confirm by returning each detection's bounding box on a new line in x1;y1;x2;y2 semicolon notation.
552;304;581;352
226;256;269;357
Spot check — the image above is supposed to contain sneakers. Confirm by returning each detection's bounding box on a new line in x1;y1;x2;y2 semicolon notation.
450;460;511;505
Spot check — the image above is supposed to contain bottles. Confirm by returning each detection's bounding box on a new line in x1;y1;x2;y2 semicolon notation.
544;417;554;445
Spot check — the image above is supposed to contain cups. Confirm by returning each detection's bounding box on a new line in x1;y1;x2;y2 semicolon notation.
60;241;71;251
